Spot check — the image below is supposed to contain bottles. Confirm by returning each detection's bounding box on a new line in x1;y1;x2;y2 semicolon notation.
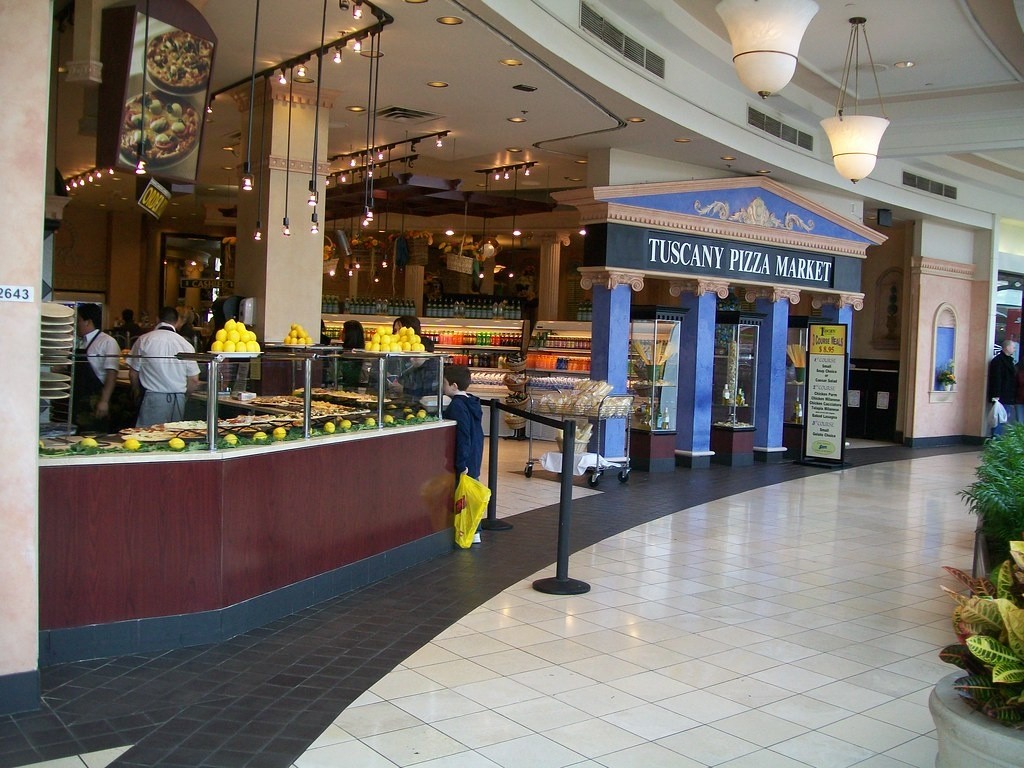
629;339;669;354
627;359;648;379
445;353;509;368
576;300;591;322
425;297;522;320
526;354;591;371
526;376;589;391
640;397;670;430
796;402;802;423
713;324;733;356
470;372;506;385
723;384;745;406
534;331;592;350
344;295;416;316
322;325;345;340
362;327;378;341
322;294;339;313
422;330;522;347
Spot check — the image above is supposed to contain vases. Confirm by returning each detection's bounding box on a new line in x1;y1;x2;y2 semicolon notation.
941;382;952;391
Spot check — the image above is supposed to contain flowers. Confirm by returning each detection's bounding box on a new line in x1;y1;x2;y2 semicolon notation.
438;241;486;287
937;359;957;386
388;230;433;246
323;243;338;262
351;233;385;253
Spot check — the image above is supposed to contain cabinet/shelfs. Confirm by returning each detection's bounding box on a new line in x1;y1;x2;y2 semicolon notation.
524;385;635;488
321;313;531;441
524;318;681;475
782;316;833;461
710;310;768;468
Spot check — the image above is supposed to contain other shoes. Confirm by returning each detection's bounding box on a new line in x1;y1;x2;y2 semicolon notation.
472;532;482;543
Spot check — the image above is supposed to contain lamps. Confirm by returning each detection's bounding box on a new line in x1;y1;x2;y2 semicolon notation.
65;0;539;282
714;0;820;100
819;17;891;185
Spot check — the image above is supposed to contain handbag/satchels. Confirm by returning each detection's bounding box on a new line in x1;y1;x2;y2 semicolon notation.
454;473;492;549
987;398;1008;428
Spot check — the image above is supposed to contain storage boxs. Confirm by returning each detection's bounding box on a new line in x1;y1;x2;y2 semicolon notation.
230;390;256;401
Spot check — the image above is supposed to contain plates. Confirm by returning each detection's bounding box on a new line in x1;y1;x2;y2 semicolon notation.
281;344;316;348
252;415;325;423
141;28;206;98
41;302;74;400
121;432;174;442
119;92;201;171
362;350;429;356
217;422;251;426
296;389;391;402
207;350;265;357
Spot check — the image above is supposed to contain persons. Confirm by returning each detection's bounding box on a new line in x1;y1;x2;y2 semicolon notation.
51;304;119;434
320;316;442;402
433;364;483;544
113;305;229;433
986;339;1020;439
1013;358;1024;426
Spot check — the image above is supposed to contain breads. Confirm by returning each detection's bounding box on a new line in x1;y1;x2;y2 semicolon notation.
534;379;633;418
556;424;593;441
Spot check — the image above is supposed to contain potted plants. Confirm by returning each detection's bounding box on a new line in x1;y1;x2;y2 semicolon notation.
929;421;1023;768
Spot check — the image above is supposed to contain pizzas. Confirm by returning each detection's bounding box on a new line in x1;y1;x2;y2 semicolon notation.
119;90;199;160
146;30;212;91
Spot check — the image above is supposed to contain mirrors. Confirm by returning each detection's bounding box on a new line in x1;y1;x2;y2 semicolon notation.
158;232;226;335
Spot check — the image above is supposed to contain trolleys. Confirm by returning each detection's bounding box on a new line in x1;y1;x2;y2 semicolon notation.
524;382;635;487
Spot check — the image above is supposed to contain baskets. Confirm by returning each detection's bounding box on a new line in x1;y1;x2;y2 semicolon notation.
555;436;590;453
504;394;530;411
505;353;527;373
502;372;527;392
505;417;526;429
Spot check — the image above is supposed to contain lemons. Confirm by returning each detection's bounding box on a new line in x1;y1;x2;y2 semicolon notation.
253;432;268;441
80;438;98;447
387;404;426;420
211;319;260;353
324;422;335;433
123;439;140;450
365;326;425;352
283;323;313;344
365;418;375;425
168;438;185;448
340;420;351;429
383;415;393;423
223;433;238;444
273;427;287;439
